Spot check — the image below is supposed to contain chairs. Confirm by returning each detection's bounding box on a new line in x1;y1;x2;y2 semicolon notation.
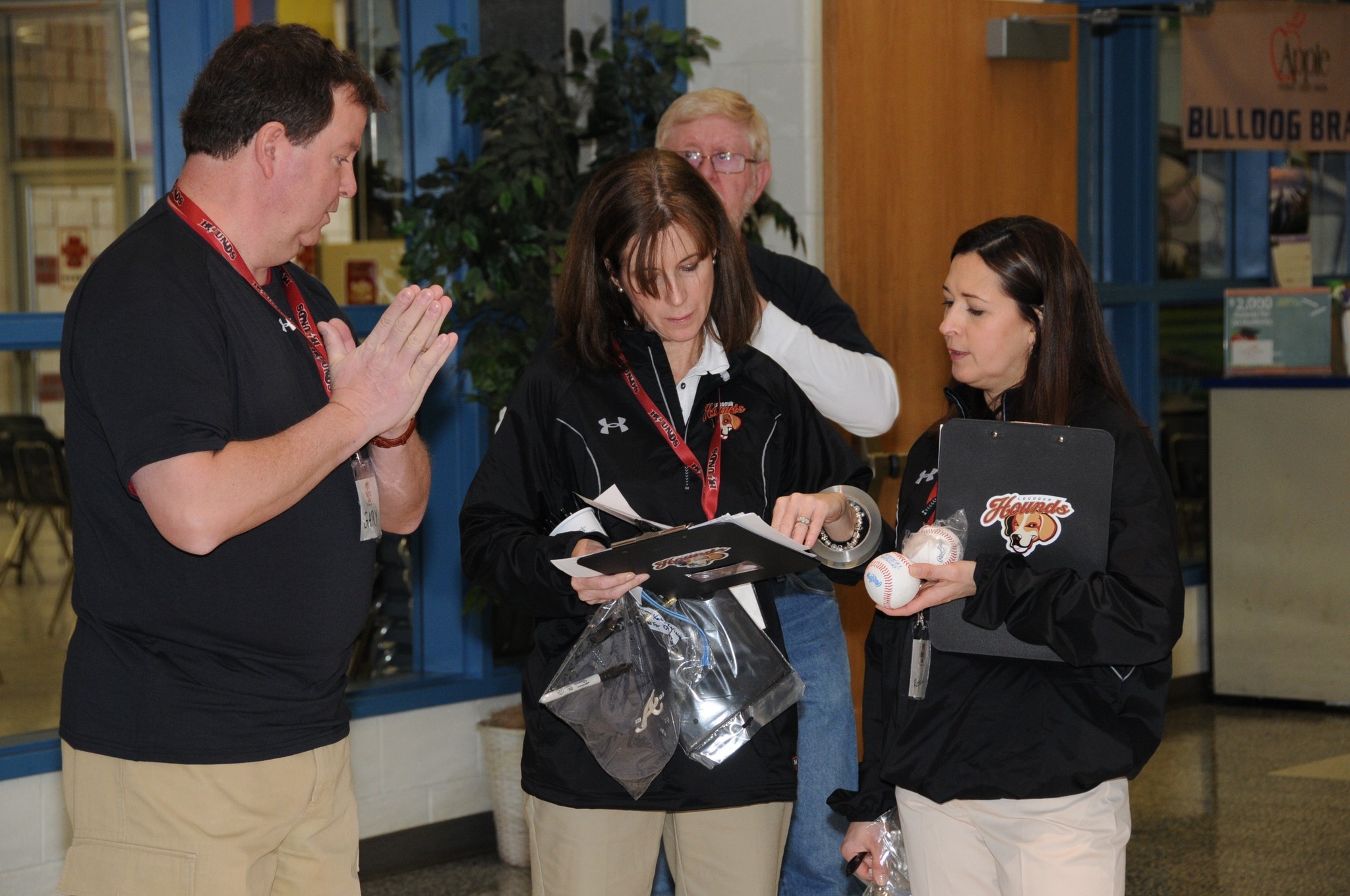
0;413;78;637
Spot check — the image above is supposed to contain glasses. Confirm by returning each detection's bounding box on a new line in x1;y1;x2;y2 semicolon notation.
673;150;756;173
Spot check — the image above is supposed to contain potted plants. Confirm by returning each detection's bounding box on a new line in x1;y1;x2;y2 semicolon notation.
402;7;810;872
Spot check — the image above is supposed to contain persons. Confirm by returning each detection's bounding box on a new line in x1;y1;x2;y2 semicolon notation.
830;217;1185;896
61;22;462;896
461;88;901;896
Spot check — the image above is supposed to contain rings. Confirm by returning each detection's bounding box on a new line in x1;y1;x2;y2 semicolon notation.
797;517;810;525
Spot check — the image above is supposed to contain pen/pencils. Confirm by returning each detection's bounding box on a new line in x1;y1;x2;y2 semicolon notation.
634;519;662;532
846;852;868;876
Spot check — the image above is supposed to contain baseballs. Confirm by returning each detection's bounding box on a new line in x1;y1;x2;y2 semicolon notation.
900;526;965;582
864;552;922;608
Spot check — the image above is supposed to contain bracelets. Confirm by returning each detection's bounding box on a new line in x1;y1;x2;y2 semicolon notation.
820;497;867;551
369;418;416;451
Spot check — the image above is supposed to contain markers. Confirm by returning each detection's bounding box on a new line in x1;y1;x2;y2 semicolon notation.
540;662;633;703
